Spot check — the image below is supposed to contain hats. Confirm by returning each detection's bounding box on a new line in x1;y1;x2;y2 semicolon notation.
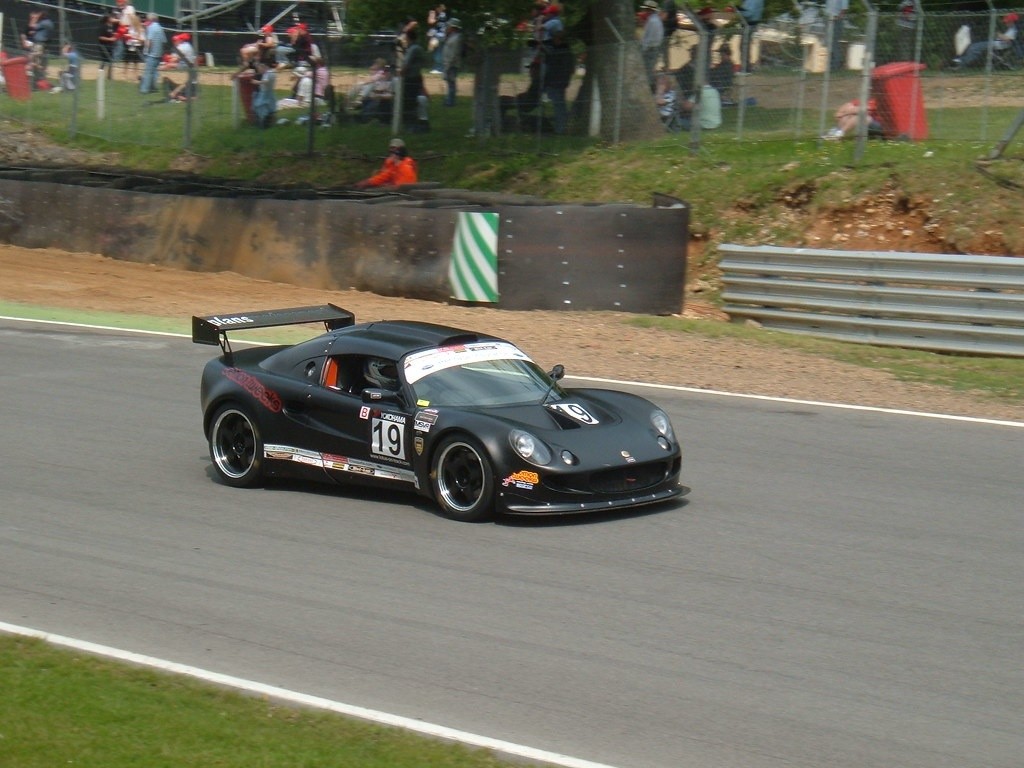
1002;13;1019;22
177;33;190;40
446;18;463;30
641;1;661;12
543;6;564;17
388;138;406;147
261;23;273;32
391;146;411;155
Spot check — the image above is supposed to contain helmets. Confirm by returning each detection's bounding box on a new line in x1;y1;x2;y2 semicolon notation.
363;357;402;393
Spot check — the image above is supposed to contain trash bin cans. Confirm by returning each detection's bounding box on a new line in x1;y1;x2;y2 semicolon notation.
0;57;31;100
868;61;929;143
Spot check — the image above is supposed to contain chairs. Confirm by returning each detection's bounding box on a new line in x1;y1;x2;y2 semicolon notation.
989;32;1021;71
322;357;353;393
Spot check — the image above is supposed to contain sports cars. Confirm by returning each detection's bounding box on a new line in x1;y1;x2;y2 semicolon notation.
193;303;691;523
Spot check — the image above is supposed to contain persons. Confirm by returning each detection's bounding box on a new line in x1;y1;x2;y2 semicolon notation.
161;64;199;101
59;42;82;91
363;359;402;391
442;18;462;108
653;44;734;129
948;14;1019;72
823;86;886;141
464;26;499;139
159;33;194;70
345;20;425;126
733;0;764;27
826;0;849;73
499;0;592;133
97;0;167;93
22;10;54;91
229;24;329;129
640;0;663;94
0;41;8;82
353;139;417;187
428;4;447;74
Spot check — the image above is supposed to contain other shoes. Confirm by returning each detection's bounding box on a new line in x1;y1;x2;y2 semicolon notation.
441;97;456;108
819;134;844;144
